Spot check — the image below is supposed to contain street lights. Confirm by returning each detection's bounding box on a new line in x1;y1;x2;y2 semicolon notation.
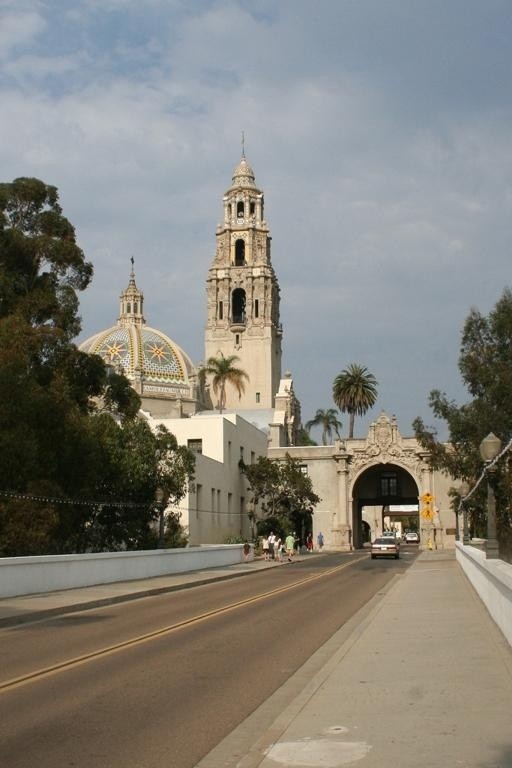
479;429;501;561
155;485;168;539
458;480;472;546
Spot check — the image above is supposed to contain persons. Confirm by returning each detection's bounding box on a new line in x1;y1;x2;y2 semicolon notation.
307;532;312;554
318;532;324;552
262;531;301;562
243;540;251;563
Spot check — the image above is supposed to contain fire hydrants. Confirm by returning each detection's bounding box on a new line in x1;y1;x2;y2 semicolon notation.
427;538;433;550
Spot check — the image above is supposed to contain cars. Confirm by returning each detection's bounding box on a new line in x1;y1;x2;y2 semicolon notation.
381;531;397;537
370;535;401;561
405;532;419;545
